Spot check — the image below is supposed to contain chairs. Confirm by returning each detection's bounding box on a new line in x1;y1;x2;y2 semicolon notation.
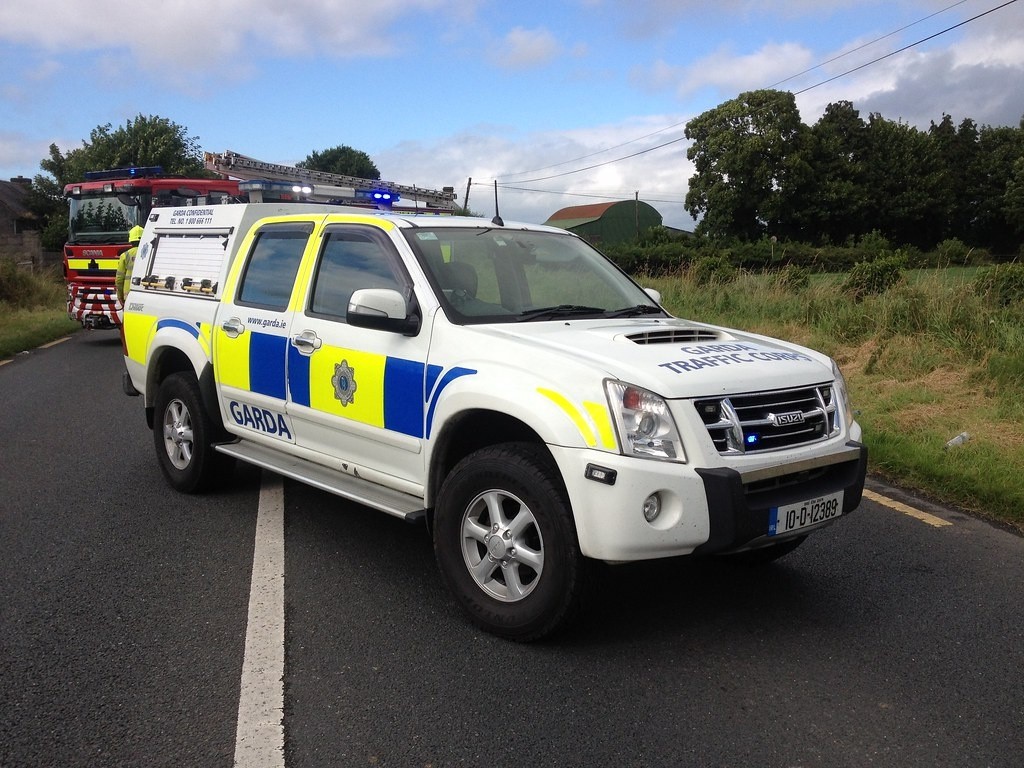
440;261;512;317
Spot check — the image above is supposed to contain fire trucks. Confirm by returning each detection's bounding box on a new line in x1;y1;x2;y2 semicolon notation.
63;150;462;342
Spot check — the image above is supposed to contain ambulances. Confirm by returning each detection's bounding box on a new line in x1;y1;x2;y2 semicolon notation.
124;184;867;641
